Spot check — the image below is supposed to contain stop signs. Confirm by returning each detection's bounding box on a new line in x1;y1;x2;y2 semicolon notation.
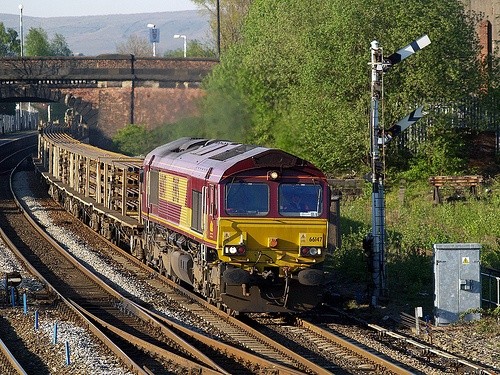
7;271;22;287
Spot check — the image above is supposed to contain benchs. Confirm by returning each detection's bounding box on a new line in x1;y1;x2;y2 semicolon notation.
429;175;484;204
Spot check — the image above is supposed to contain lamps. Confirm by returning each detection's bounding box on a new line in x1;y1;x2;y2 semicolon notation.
6;271;22;286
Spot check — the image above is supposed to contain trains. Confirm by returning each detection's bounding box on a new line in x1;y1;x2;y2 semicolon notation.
30;122;342;316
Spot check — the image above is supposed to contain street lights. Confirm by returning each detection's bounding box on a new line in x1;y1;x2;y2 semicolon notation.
174;34;187;58
17;4;24;57
147;24;156;57
